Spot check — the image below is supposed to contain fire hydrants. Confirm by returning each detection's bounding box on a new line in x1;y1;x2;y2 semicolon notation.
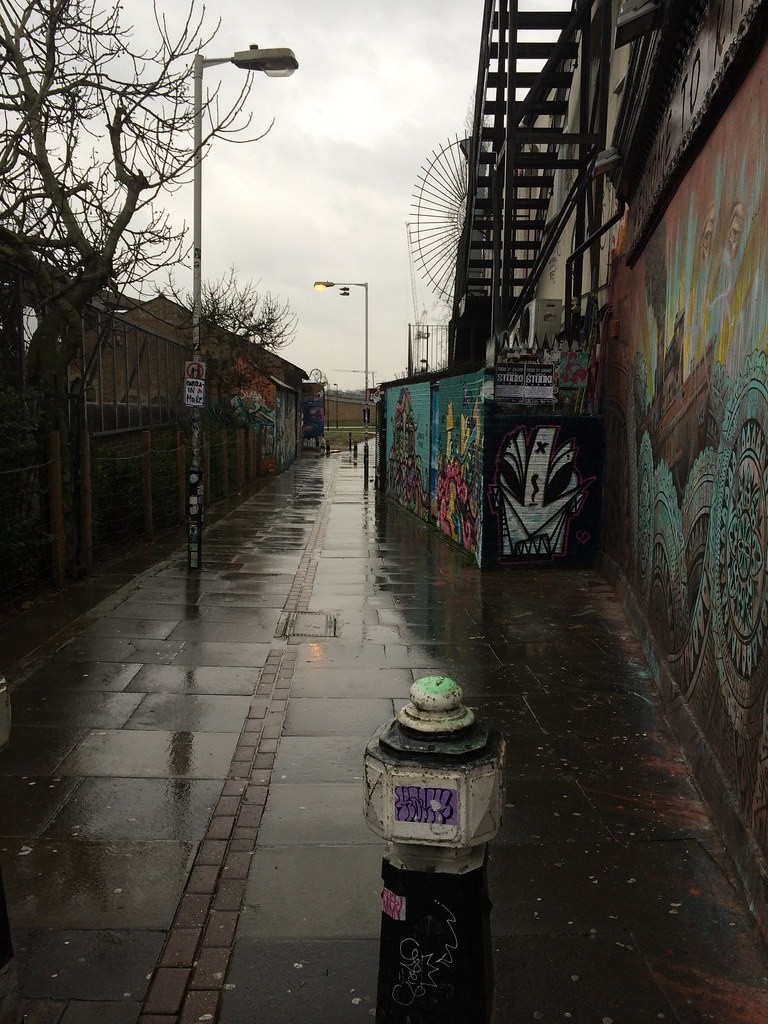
359;675;499;1024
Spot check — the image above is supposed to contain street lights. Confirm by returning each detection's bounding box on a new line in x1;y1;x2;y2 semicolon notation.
314;280;370;463
333;383;339;428
185;41;300;572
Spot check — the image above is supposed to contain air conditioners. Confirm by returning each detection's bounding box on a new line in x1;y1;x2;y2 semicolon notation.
519;298;562;351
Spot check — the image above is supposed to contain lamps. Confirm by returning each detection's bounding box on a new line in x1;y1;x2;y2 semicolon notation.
588;147;623;178
